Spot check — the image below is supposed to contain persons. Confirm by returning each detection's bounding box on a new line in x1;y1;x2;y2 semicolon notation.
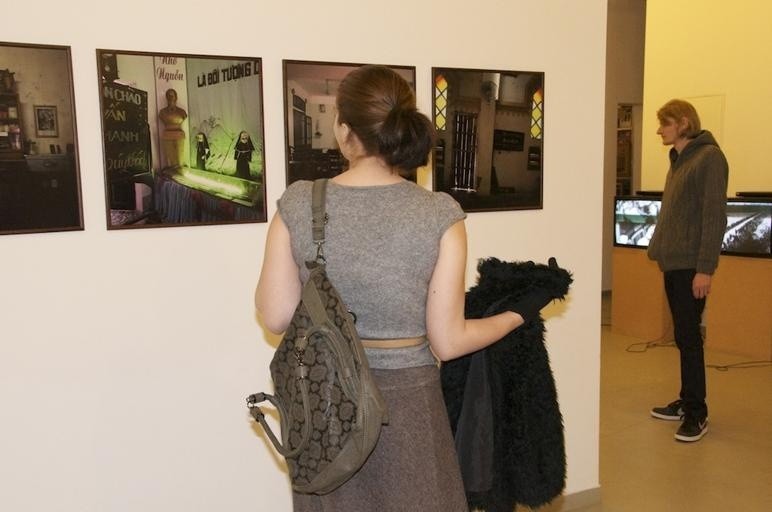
157;87;188;166
646;97;730;444
253;62;573;512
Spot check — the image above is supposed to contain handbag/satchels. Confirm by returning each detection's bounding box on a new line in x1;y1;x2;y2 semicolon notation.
245;256;388;494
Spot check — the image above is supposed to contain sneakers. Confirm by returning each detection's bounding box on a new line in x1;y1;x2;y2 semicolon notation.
674;417;709;442
651;400;685;420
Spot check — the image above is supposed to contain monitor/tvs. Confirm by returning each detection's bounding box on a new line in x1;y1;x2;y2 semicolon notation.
717;199;772;259
612;195;664;250
